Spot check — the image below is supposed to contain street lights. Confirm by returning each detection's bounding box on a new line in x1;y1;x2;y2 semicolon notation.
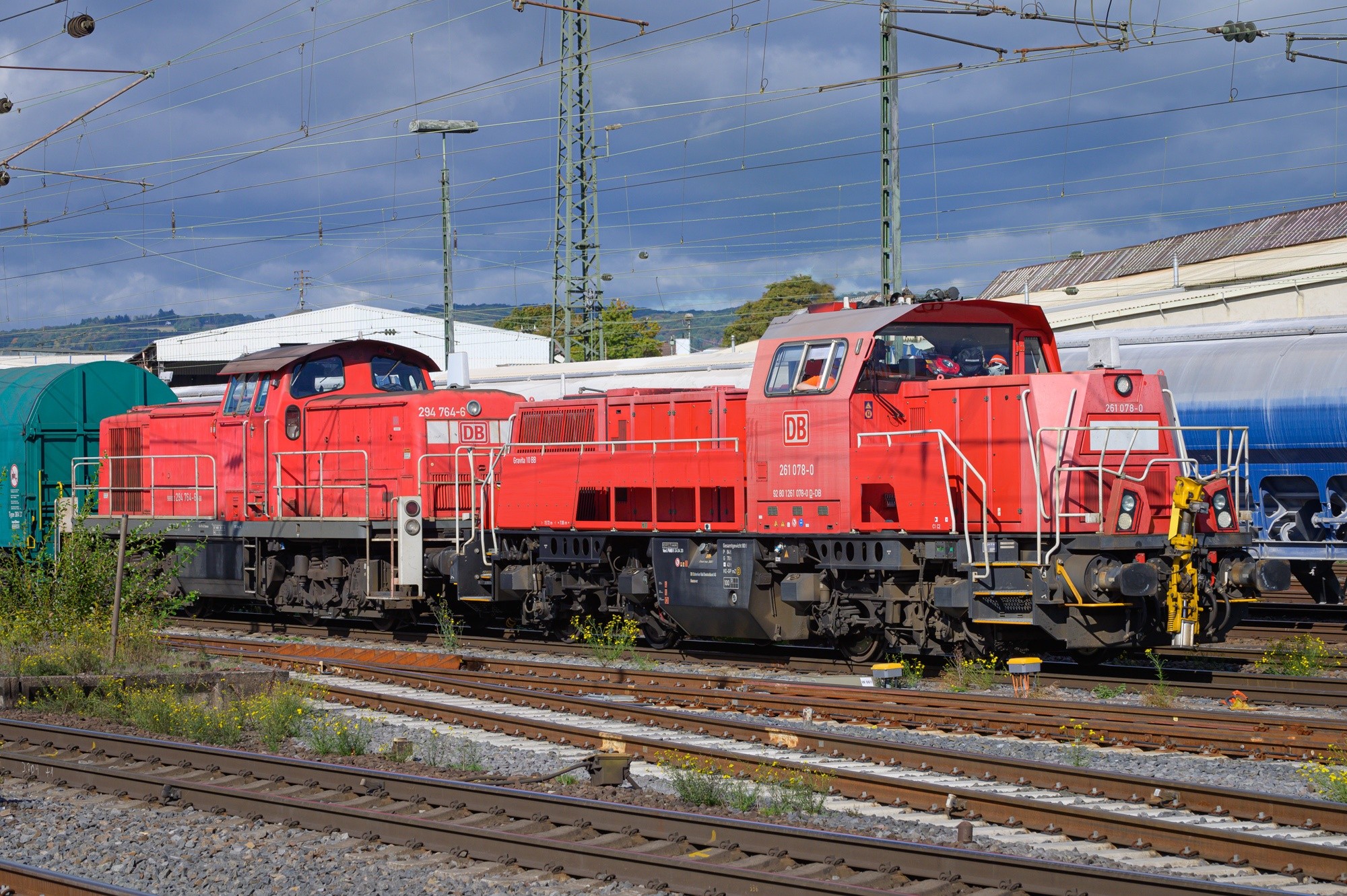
409;119;479;371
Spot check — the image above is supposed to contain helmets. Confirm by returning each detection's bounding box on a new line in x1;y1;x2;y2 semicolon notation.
989;354;1009;366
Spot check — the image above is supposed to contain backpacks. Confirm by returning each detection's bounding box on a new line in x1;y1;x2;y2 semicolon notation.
955;348;983;375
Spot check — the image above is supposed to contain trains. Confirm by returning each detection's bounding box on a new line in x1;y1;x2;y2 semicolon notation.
0;295;1268;667
1022;315;1347;603
